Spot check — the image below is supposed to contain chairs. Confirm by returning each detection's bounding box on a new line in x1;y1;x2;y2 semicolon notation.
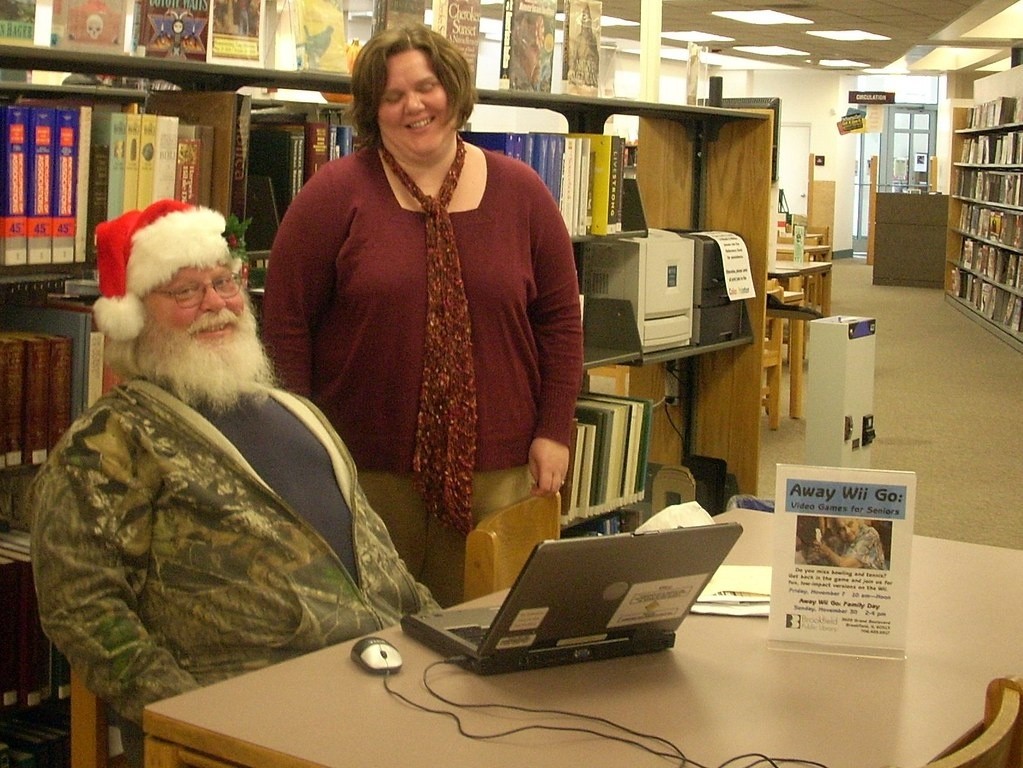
71;667;126;768
465;490;561;601
926;675;1023;768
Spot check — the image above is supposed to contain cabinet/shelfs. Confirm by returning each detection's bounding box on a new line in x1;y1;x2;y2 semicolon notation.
0;42;774;768
944;107;1023;353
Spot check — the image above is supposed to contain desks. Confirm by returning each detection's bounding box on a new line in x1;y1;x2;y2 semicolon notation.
143;508;1023;768
762;233;833;431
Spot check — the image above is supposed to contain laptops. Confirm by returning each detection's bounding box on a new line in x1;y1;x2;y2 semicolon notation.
400;521;743;677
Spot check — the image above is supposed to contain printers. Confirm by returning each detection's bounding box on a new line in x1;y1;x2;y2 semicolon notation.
581;231;743;354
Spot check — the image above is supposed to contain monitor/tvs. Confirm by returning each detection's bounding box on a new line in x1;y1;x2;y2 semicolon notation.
704;97;782;183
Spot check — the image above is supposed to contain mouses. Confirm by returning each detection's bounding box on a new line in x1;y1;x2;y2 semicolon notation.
350;637;402;675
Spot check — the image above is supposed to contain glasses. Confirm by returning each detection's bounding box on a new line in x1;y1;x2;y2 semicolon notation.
151;272;245;309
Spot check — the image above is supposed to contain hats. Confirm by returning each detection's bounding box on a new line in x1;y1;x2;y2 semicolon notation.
92;199;226;342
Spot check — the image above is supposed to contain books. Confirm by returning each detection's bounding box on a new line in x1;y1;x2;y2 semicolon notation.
291;0;350;76
951;266;1023;332
0;527;71;768
961;131;1023;164
0;71;362;276
2;0;267;69
958;235;1023;292
0;280;125;472
369;0;426;39
432;0;482;90
459;129;625;238
965;97;1016;127
562;0;602;89
954;167;1023;207
957;200;1023;248
561;393;653;517
498;0;557;93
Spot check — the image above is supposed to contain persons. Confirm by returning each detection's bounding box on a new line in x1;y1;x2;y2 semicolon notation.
813;518;887;570
27;198;443;728
259;24;584;607
